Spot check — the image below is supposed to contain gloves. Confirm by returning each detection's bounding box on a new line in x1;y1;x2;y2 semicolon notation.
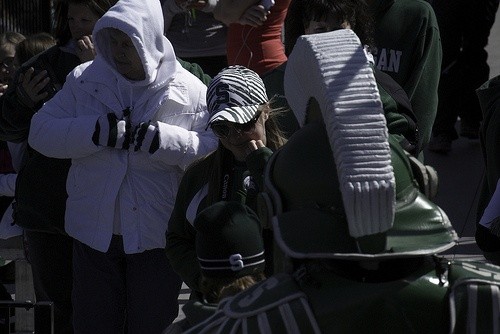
130;121;162;155
91;112;127;149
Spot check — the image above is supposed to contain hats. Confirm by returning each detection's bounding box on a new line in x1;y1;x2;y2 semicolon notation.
193;201;265;278
203;65;266;131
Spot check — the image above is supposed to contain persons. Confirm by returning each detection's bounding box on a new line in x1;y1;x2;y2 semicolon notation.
419;0;500;157
165;64;288;303
472;75;500;269
475;180;500;254
157;200;273;334
353;1;444;170
0;29;57;271
64;0;216;94
175;110;499;333
158;0;229;88
213;0;298;145
0;0;82;334
258;0;428;180
28;0;223;334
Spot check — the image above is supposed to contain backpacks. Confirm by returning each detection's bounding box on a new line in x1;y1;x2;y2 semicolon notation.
10;144;74;236
